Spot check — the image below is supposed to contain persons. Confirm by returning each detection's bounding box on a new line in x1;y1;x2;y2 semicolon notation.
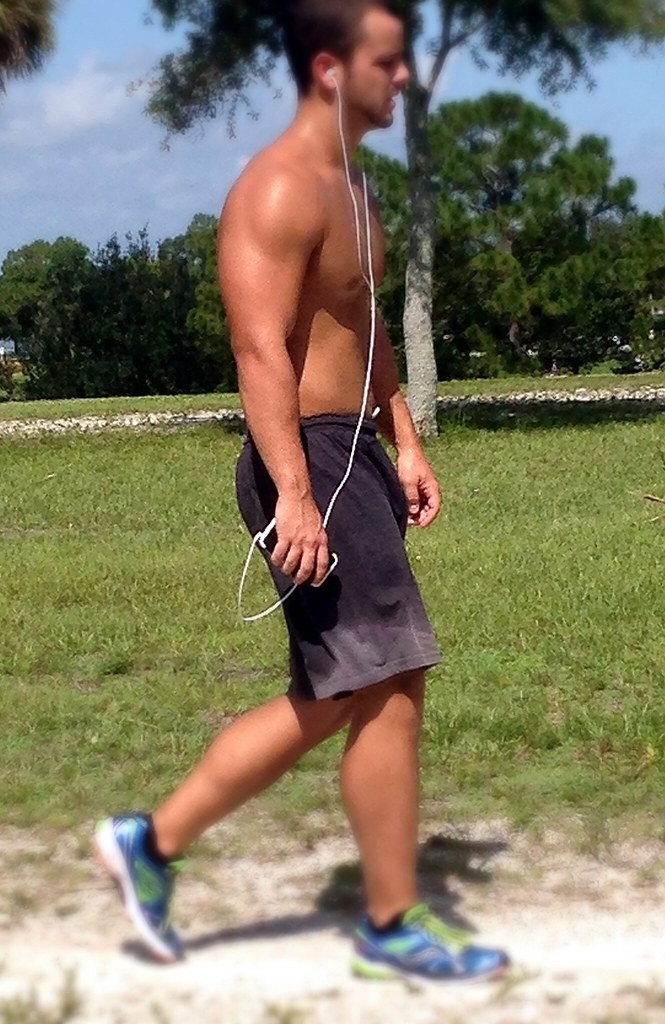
87;0;512;986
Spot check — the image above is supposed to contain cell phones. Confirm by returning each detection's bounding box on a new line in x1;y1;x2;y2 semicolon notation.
259;517;338;588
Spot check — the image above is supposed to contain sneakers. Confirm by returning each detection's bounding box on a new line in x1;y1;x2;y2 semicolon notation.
94;814;186;962
355;904;508;989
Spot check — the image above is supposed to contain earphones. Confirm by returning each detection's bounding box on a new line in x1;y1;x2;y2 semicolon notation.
327;68;342;81
